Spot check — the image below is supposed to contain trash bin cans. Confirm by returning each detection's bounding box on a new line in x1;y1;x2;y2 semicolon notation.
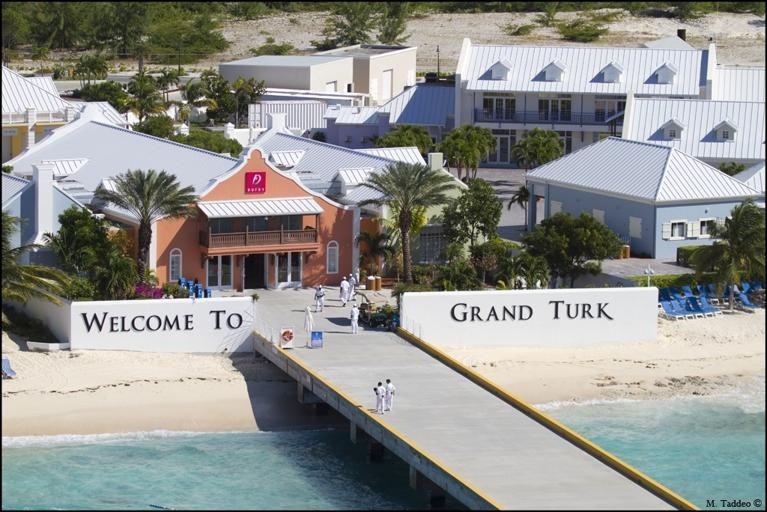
187;280;194;295
204;288;211;298
195;285;202;298
180;278;186;287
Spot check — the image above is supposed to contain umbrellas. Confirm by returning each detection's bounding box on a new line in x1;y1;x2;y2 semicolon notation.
303;306;314;347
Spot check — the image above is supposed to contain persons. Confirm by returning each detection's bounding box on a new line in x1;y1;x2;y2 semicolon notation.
385;379;395;411
349;273;356;301
375;381;386;414
315;286;326;312
340;276;350;307
351;303;359;334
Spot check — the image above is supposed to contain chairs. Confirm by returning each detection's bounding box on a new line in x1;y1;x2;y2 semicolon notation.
659;280;766;321
